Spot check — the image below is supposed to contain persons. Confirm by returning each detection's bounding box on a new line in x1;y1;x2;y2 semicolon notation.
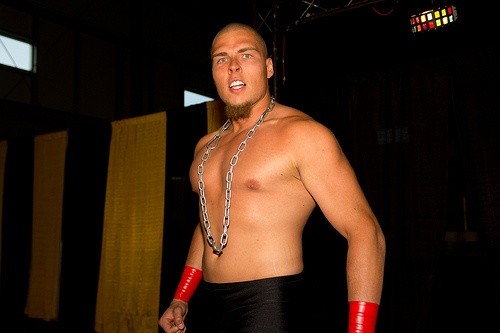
157;24;387;333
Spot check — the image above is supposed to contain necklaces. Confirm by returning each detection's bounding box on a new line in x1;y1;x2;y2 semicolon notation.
196;95;275;256
